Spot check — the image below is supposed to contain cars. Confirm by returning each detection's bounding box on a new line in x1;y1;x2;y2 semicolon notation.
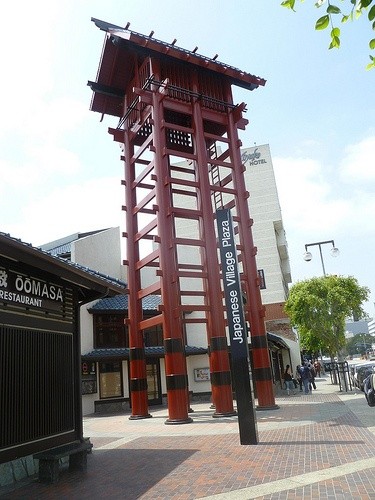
315;350;375;407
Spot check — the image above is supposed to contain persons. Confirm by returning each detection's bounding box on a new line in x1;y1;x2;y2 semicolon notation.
308;364;316;391
298;362;309;395
310;361;315;371
296;365;303;391
284;364;296;396
314;360;321;378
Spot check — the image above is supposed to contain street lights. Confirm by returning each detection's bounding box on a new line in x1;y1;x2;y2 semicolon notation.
302;240;340;274
